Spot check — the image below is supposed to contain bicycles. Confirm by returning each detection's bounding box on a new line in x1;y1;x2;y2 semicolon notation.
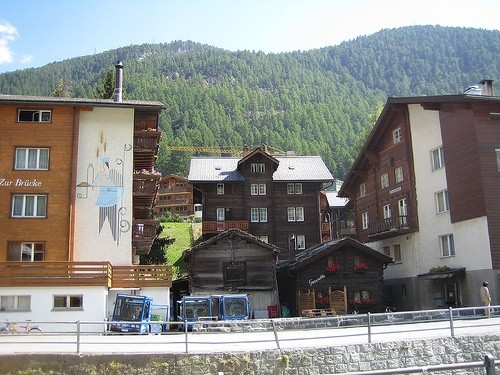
1;319;43;336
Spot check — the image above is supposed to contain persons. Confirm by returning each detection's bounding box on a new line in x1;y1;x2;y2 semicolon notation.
479;281;493;320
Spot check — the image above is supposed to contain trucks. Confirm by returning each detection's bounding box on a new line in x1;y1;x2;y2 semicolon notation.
176;296;213;331
110;294;165;333
210;296;249;321
